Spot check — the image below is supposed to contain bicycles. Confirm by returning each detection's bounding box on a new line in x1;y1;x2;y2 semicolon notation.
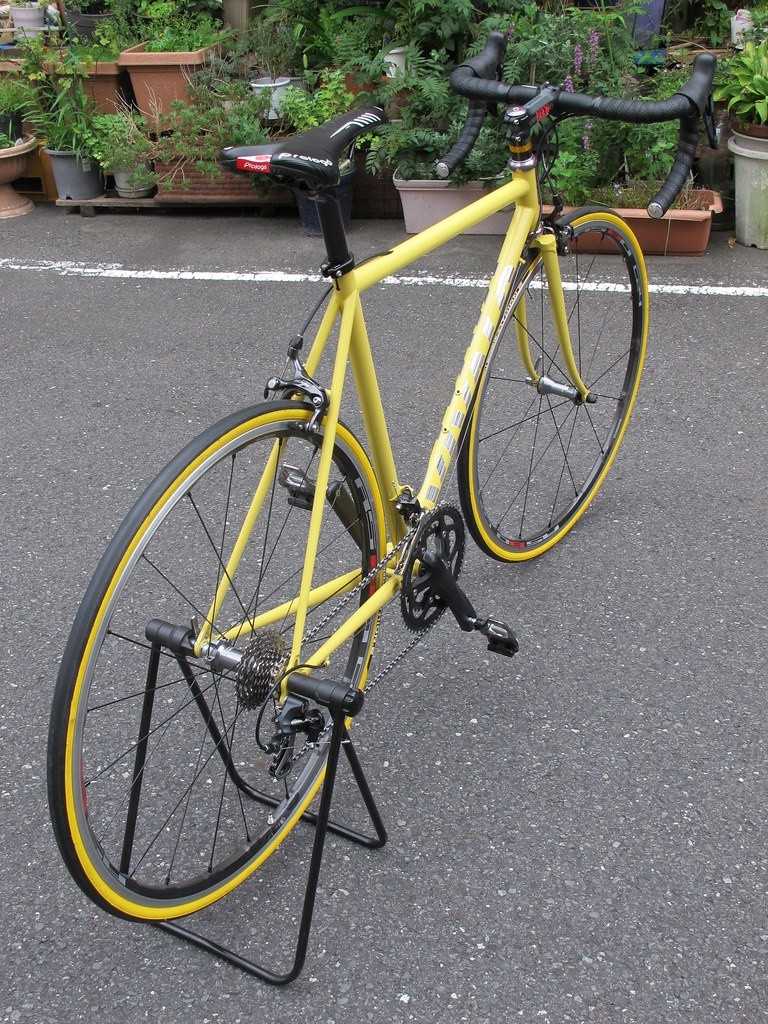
42;31;731;991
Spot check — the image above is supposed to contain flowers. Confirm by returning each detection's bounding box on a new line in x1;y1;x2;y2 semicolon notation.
480;20;599;158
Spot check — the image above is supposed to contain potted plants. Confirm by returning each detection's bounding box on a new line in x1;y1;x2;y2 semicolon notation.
0;0;768;257
